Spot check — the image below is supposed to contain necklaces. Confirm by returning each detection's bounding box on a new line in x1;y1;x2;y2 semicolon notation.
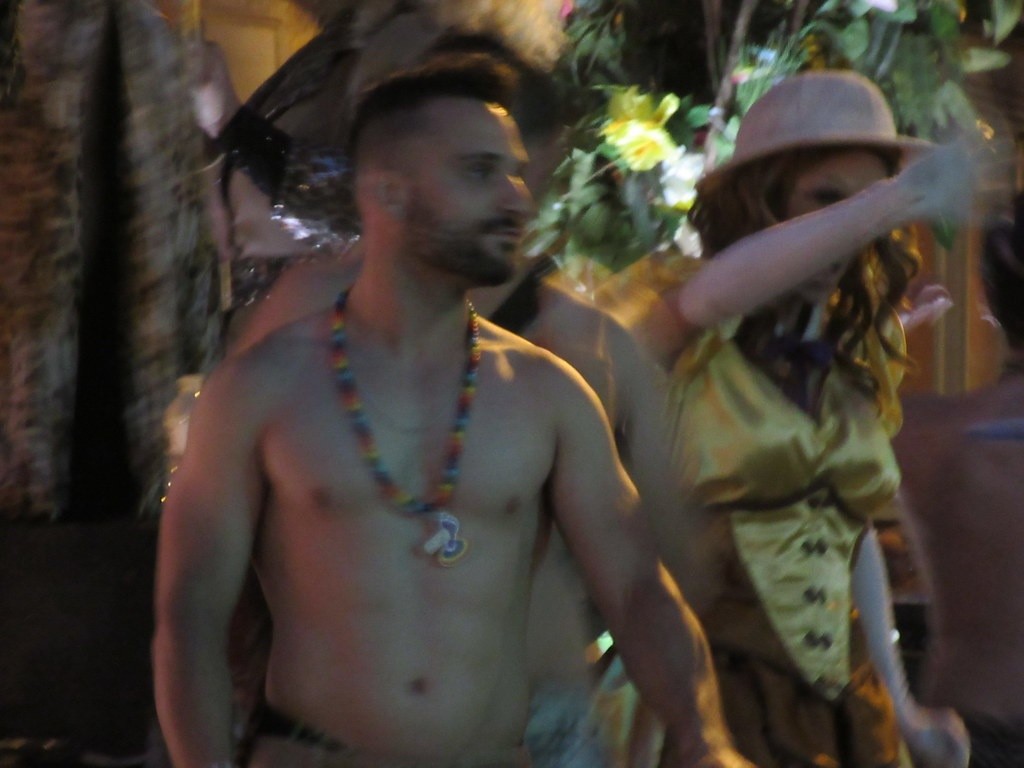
329;284;480;564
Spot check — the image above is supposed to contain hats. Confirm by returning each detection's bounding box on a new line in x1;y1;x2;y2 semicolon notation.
693;72;934;195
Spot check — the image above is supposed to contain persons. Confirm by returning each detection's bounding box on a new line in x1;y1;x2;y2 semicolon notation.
148;54;761;768
888;186;1023;768
580;70;1024;768
155;0;715;641
